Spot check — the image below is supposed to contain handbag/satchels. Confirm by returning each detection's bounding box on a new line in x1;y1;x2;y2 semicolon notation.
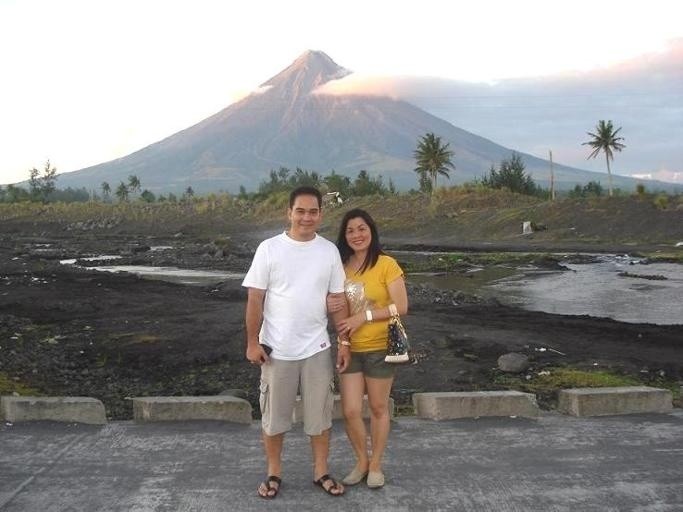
385;318;415;364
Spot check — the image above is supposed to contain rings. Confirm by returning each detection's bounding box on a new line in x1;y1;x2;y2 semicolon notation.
347;327;350;330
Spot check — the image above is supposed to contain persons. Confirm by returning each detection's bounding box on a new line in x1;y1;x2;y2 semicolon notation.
325;207;409;489
239;185;353;501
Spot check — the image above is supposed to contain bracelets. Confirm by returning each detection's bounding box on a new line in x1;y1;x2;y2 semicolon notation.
364;310;372;322
340;340;351;346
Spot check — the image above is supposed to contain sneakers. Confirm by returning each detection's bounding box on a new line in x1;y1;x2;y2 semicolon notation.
367;469;385;488
342;461;369;486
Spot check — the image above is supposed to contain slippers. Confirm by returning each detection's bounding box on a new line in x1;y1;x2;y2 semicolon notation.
313;474;345;496
257;476;283;500
